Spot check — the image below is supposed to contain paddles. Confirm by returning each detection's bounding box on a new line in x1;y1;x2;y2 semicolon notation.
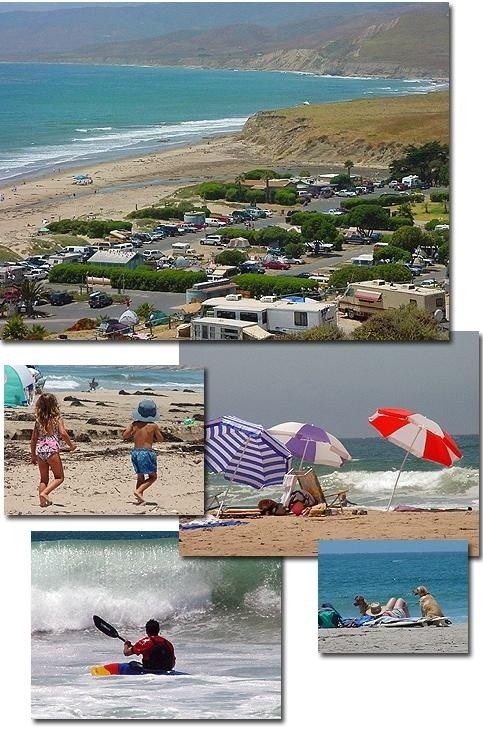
93;615;135;648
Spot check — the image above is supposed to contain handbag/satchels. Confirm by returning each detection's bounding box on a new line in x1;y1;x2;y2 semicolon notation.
320;604;343;628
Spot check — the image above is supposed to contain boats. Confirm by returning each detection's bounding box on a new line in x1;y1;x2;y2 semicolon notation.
92;663;191;677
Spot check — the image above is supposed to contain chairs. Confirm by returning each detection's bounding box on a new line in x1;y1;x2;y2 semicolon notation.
206;491;262;519
285;466;351;516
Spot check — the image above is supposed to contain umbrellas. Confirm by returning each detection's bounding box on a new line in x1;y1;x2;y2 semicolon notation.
366;408;465;513
205;414;293;518
263;421;352;472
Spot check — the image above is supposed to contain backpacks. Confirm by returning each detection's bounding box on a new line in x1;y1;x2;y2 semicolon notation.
148;635;171;670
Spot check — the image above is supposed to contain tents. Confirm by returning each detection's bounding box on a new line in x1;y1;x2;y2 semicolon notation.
2;365;35;410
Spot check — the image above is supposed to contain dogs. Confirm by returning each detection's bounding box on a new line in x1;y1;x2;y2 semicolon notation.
412;586;443;627
352;595;371;616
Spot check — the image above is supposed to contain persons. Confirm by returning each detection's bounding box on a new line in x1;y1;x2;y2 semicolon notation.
122;399;163;505
90;378;96;391
318;602;341;629
365;597;408;620
25;365;46;406
30;393;77;507
122;618;176;674
334;494;357;506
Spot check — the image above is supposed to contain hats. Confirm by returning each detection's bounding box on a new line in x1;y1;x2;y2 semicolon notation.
132;400;161;422
365;602;385;616
